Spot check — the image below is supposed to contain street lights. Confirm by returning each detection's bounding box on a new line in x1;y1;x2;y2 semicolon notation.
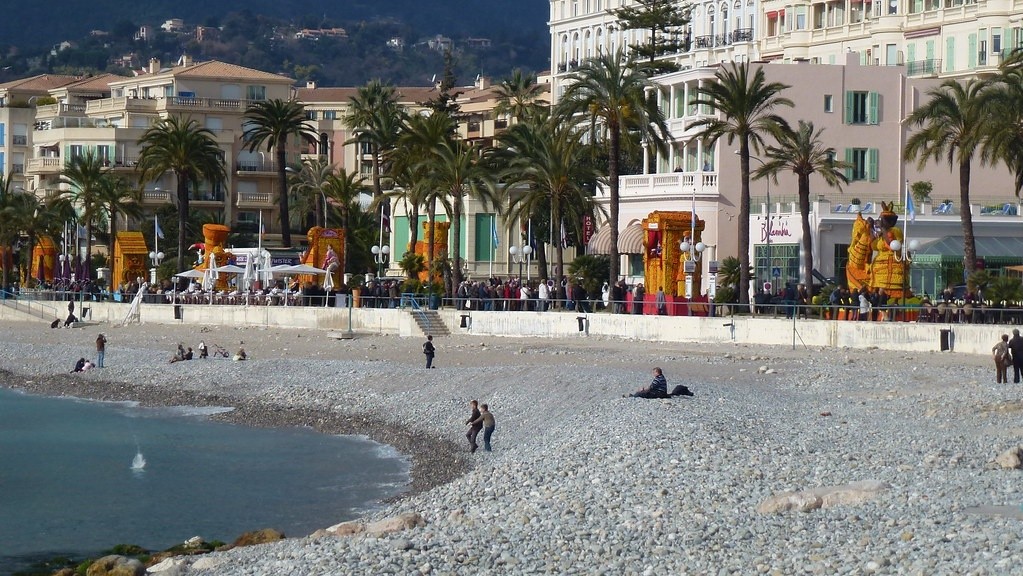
507;147;553;308
155;188;181;275
371;246;390;296
15;186;50;229
149;252;164;291
285;167;326;227
509;246;532;288
680;242;705;315
890;240;920;321
735;150;770;303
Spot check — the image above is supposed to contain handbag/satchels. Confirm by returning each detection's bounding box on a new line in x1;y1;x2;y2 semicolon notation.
201;346;207;355
198;343;205;350
1004;353;1011;367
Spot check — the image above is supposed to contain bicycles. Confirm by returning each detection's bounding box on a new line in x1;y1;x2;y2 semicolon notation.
211;344;229;358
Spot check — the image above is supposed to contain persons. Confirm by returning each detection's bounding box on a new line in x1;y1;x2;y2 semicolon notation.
1009;329;1023;383
751;282;970;322
468;404;495;453
423;336;435;369
703;160;713;182
655;286;665;314
465;400;483;454
10;277;400;329
69;334;246;373
623;367;666;399
674;166;683;172
991;334;1009;383
455;274;644;316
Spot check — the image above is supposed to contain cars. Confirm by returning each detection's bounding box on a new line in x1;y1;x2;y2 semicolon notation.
935;292;956;304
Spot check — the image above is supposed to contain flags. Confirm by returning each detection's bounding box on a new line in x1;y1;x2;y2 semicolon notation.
76;223;96;240
526;220;535;250
381;206;391;232
560;218;566;249
905;189;914;222
489;217;498;249
155;219;164;239
260;216;264;234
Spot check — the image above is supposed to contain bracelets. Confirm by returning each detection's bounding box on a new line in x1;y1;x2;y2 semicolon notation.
643;388;643;391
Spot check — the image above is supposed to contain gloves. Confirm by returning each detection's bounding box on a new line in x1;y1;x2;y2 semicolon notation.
465;421;469;425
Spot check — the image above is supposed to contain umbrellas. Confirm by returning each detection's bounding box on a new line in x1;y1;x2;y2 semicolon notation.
37;252;89;281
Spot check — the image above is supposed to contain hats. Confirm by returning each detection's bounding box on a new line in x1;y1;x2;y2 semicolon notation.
1013;329;1019;334
81;358;85;362
1002;335;1008;340
99;334;103;338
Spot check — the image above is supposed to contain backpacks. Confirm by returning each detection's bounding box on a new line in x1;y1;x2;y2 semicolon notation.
830;289;838;304
422;342;430;354
672;385;694;396
